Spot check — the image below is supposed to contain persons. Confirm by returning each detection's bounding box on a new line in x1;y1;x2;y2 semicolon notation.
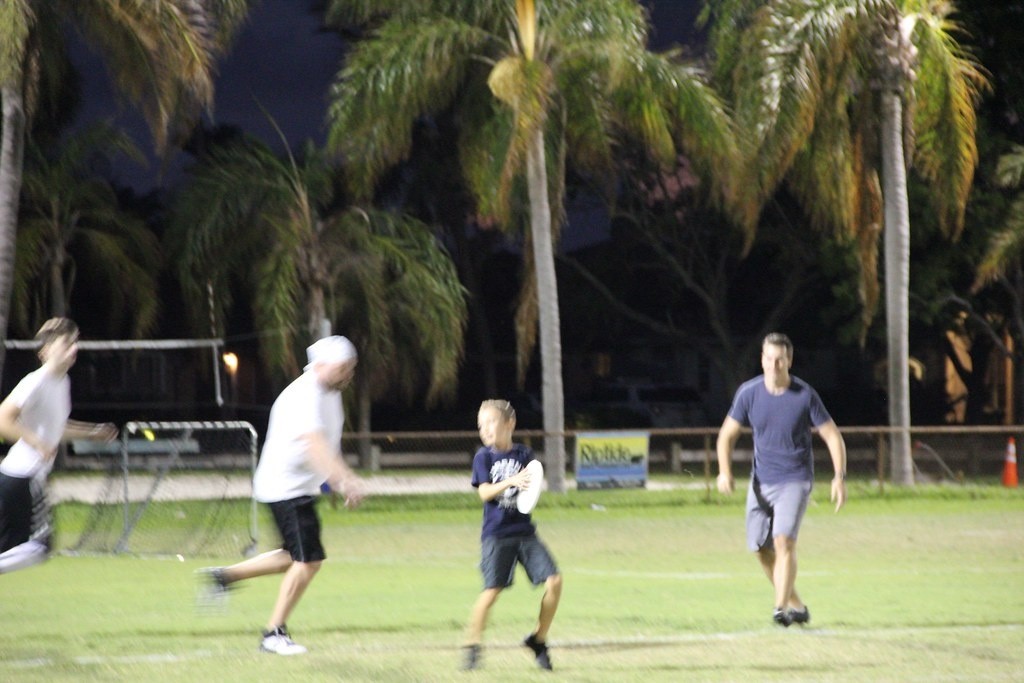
199;337;366;657
716;332;847;628
459;400;562;673
0;316;117;573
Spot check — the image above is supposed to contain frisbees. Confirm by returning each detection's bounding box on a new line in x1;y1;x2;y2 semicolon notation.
516;458;544;515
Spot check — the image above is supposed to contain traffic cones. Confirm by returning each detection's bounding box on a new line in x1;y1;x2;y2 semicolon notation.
1000;436;1018;488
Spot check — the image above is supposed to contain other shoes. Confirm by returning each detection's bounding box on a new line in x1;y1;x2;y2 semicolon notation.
524;635;553;672
260;629;307;655
459;644;479;671
773;604;809;626
